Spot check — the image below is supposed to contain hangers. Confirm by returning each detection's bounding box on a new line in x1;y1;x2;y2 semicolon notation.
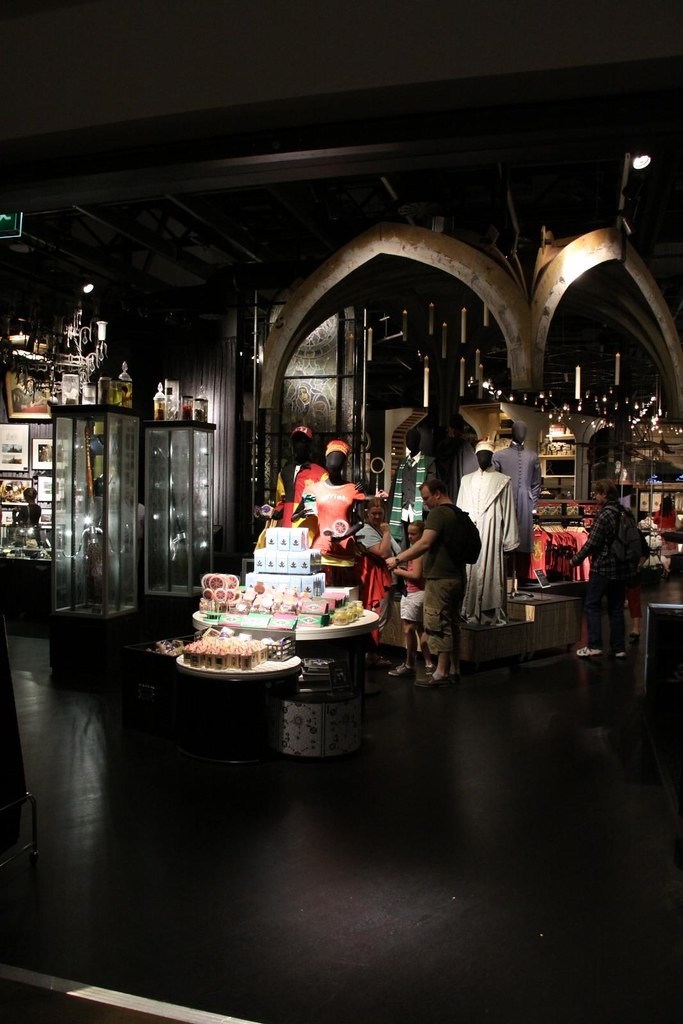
529;513;590;537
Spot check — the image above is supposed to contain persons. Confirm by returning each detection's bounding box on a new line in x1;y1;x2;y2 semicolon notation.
384;478;468;688
652;497;676;577
355;497;405;668
383;428;441;594
253;426;323;524
12;489;41;529
77;477;145;608
432;414;474;505
388;520;436;677
570;478;628;658
623;509;651;638
291;438;366;587
453;440;522;626
494;420;541;584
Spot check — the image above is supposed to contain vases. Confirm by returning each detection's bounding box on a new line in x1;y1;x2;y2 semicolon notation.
0;477;33;508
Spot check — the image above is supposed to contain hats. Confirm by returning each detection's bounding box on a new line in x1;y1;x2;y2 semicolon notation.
290;426;313;441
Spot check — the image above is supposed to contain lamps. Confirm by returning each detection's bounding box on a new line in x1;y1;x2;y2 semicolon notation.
78;275;94;294
17;304;30;322
631;149;652;170
48;297;109;392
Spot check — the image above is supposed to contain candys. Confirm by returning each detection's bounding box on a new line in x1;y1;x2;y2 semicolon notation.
321;519;350;538
200;573;239;604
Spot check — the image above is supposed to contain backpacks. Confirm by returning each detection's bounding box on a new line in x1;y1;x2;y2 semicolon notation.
603;505;651;564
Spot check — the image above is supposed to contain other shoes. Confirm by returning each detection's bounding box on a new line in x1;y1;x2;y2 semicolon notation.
614;649;627;657
628;631;640;642
576;646;607;656
369;652;392;668
447;671;462;684
414;674;450;688
424;664;437;675
387;663;415;677
661;568;671;580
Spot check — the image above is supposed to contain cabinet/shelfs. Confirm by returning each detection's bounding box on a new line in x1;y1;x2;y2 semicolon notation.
538;434;576;474
140;419;217;646
46;402;146;694
378;589;581;675
537;499;603;528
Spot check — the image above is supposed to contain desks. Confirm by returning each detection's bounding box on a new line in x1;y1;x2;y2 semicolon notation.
169;652;302;765
191;605;380;730
261;678;364;764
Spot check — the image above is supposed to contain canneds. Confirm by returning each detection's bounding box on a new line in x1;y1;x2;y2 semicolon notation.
333;600;364;626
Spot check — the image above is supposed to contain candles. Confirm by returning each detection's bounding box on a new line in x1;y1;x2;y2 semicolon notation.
423;356;428;367
367;327;373;362
574;365;581;400
442;322;447;359
403;310;407;341
423;366;429;407
615;352;620;386
461;307;467;344
484;302;489;326
459;357;465;398
478;364;483;400
475;348;480;381
429;302;434;335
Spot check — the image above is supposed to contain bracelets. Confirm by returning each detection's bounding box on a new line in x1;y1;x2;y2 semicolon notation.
395;556;399;565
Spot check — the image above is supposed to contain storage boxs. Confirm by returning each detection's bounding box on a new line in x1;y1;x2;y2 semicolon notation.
248;525;359;604
120;634;196;740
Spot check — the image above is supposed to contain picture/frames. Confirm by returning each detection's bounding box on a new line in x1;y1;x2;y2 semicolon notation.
37;508;53;526
0;423;30;473
38;475;66;502
0;510;21;527
32;437;70;471
2;359;57;424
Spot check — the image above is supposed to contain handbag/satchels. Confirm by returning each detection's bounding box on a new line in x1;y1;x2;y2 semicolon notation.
445;503;482;564
13;506;41;544
